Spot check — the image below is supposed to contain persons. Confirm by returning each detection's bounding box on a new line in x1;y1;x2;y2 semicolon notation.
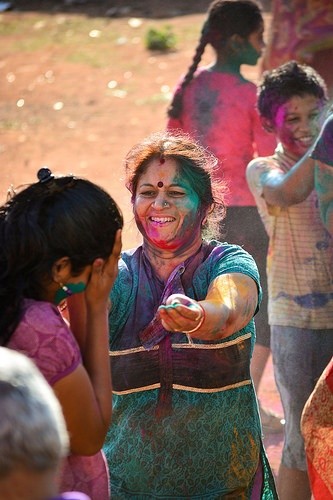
258;0;333;101
314;112;333;234
246;59;332;500
0;169;126;500
83;131;275;500
163;0;283;433
1;349;92;500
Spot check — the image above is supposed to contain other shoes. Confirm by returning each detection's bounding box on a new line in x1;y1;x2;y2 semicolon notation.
257;399;285;432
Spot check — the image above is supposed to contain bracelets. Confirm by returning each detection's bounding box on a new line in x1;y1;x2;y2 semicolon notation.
180;305;206;334
